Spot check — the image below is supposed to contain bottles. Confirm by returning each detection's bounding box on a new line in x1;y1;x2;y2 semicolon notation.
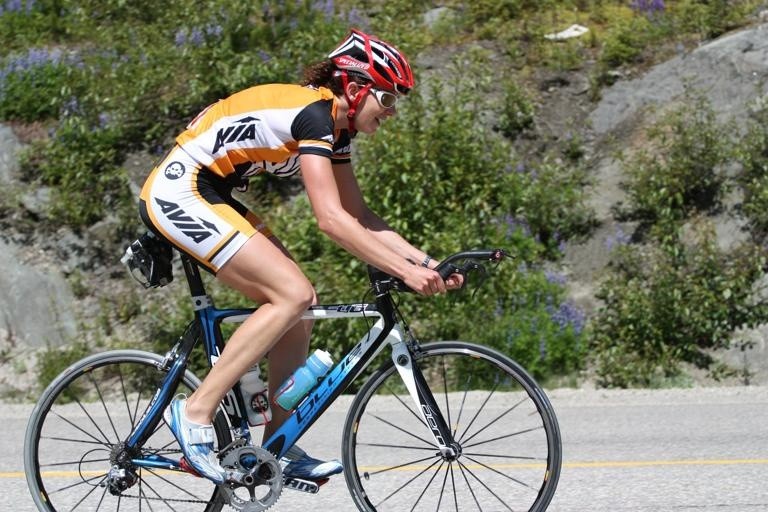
239;363;273;427
275;347;334;414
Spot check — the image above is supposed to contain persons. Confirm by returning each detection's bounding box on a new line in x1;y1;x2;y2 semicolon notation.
138;24;465;486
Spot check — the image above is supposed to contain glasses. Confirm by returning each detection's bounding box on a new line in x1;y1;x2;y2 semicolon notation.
358;84;401;110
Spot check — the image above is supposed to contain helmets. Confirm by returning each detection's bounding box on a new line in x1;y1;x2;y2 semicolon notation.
327;28;414;98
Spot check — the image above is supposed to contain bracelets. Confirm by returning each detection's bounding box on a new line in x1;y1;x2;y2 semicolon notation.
421;254;431;269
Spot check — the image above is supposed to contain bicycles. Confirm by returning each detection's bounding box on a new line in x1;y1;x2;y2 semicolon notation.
24;226;565;511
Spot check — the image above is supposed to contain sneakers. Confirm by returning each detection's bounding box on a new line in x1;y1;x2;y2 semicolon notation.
279;444;344;481
163;398;227;486
